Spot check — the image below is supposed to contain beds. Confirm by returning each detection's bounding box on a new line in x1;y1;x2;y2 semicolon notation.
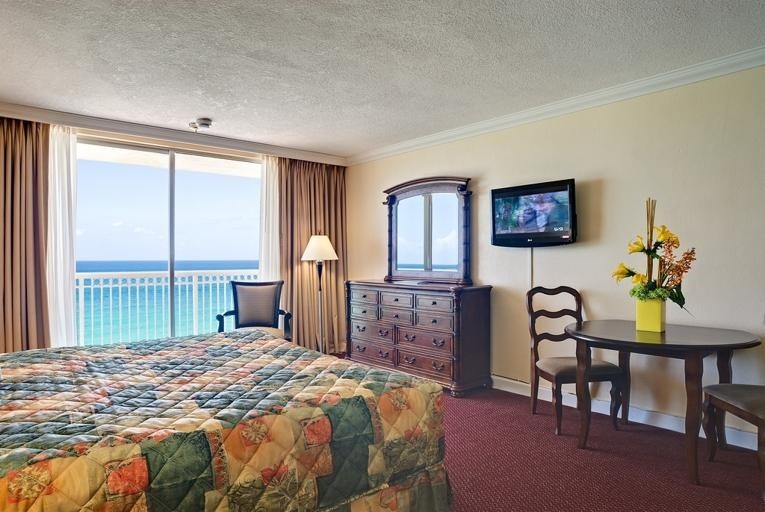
0;329;453;512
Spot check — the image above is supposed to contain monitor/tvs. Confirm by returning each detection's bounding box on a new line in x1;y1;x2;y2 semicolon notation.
490;178;578;247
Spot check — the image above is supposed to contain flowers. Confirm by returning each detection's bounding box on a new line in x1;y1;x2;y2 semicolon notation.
609;197;697;309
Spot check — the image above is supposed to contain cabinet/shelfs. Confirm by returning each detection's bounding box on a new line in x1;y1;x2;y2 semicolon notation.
343;280;492;398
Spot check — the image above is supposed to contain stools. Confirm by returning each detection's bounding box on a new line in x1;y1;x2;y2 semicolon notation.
701;383;765;503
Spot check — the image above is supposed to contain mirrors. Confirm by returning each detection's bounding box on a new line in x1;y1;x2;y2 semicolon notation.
381;176;473;285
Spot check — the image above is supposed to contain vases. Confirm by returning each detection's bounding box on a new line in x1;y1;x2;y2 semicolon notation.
635;296;667;333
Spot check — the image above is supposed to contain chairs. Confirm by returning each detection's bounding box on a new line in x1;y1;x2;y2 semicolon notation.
525;286;624;436
216;279;293;343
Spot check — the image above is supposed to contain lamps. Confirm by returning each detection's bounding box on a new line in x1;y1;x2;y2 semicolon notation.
301;235;338;355
